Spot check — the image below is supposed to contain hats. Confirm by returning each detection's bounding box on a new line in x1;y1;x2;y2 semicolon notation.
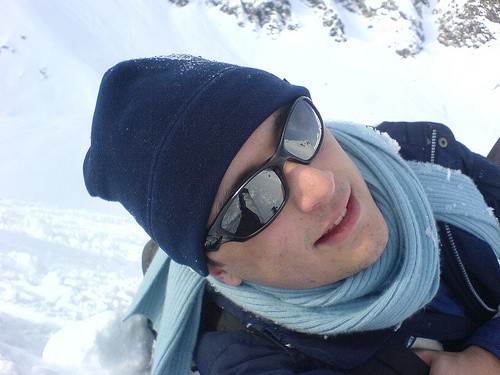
82;53;312;276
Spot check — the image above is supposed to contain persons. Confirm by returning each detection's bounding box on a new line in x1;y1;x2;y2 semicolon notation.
82;52;500;375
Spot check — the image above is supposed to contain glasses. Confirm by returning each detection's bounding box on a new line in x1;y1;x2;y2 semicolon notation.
204;96;324;252
